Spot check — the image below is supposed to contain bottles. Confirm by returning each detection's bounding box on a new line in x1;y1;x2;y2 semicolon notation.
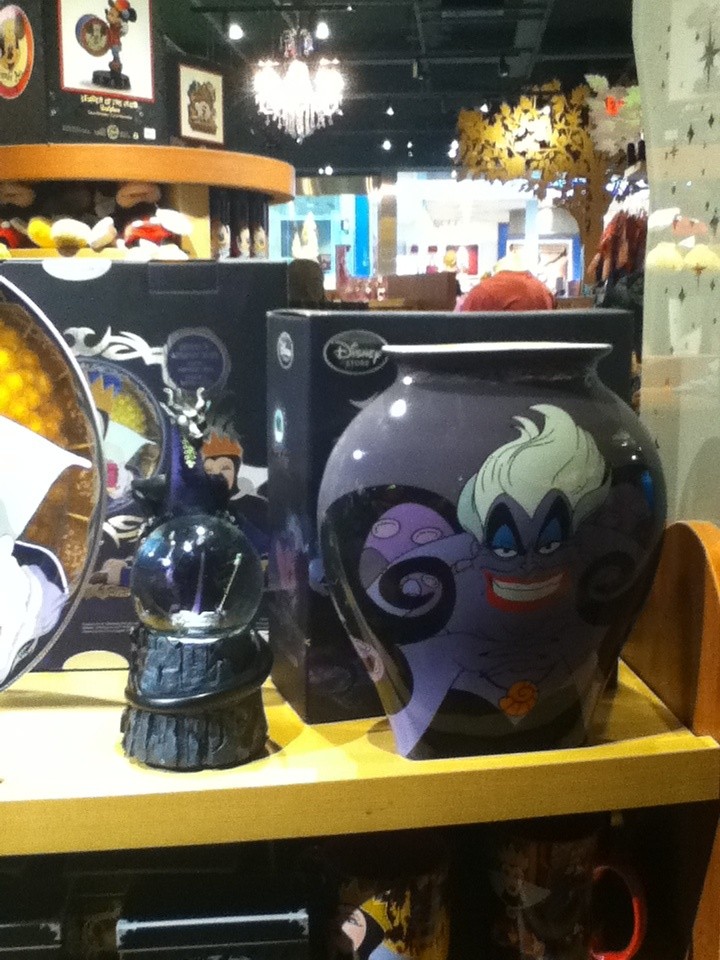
316;341;668;761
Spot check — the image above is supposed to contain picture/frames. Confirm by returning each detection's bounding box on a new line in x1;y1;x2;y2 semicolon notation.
56;1;154;104
178;64;225;147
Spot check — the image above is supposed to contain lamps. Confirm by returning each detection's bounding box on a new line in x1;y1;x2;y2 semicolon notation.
247;9;350;145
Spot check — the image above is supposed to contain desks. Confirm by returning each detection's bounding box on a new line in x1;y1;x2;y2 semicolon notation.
1;650;719;960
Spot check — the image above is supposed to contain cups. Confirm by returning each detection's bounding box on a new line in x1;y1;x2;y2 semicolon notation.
490;810;648;960
321;829;450;960
